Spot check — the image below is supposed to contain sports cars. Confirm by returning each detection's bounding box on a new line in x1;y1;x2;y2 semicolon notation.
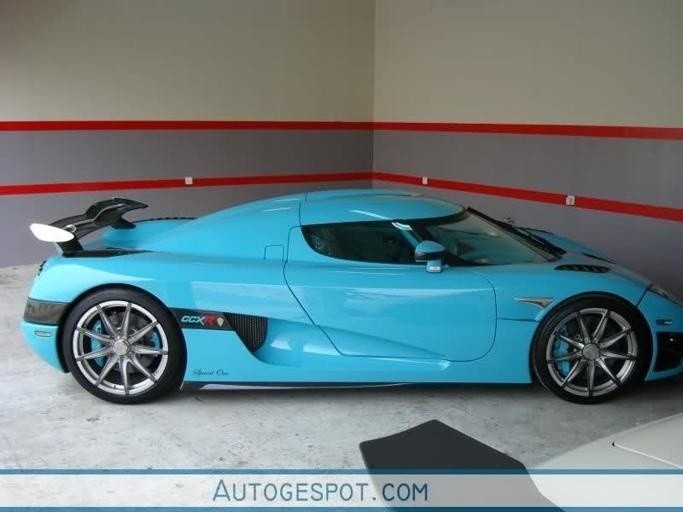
18;188;682;402
